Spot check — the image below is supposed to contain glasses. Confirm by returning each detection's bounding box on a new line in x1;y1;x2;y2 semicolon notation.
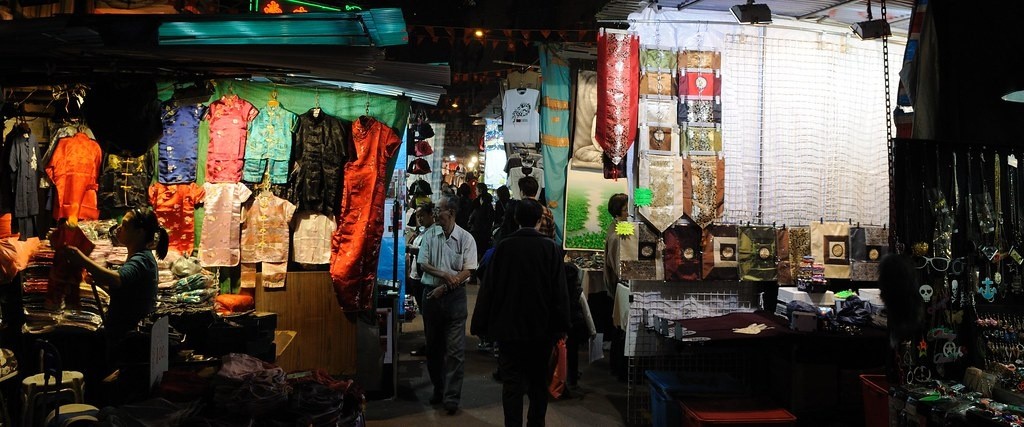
912;254;950;272
431;207;450;214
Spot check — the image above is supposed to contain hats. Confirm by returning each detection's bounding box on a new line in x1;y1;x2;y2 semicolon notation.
409;122;433;226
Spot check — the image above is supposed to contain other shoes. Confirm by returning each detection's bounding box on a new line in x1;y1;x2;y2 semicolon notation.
445;400;458;412
467;277;478;284
430;392;445;404
492;369;502;380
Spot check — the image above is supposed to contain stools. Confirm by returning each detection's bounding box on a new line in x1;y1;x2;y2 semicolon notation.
21;370;86;427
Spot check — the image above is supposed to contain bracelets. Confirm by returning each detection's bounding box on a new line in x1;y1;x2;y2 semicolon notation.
444;284;447;294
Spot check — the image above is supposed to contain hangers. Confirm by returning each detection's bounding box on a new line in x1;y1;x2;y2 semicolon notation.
260;184;270;198
312;97;320;114
13;112;85;136
361;104;369;121
66;201;80;227
225;85;237;99
517;79;526;91
520;149;535;169
598;20;726;162
268;91;280;107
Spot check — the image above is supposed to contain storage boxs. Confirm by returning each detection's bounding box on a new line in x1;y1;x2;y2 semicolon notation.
645;369;797;427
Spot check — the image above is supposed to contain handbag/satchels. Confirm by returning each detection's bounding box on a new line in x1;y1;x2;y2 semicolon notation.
547;337;569;399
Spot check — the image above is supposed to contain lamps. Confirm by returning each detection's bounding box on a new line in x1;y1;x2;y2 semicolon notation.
848;0;893;40
728;0;771;26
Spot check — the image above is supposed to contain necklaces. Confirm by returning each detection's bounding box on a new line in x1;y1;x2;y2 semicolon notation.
903;150;1024;307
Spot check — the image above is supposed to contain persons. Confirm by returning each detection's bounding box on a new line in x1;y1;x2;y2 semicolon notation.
47;232;82;309
470;198;573;427
406;204;434;354
65;206;169;407
752;280;778;313
416;195;478;411
562;262;597;398
603;194;642;384
441;176;556;283
477;226;501;357
878;255;985;385
